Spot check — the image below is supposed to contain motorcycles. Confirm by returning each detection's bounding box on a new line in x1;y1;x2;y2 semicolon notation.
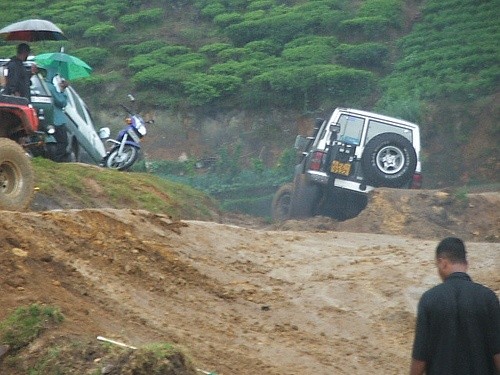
99;94;156;170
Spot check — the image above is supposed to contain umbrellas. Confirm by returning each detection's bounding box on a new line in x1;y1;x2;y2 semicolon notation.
34;53;92;83
0;19;69;46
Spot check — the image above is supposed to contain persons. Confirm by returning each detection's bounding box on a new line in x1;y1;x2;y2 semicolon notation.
410;237;500;375
44;78;69;162
7;43;37;103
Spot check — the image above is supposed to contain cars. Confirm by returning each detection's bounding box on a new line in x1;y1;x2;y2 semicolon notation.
0;55;111;166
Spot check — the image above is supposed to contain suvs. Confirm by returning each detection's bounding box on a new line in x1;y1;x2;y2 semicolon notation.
270;105;423;224
